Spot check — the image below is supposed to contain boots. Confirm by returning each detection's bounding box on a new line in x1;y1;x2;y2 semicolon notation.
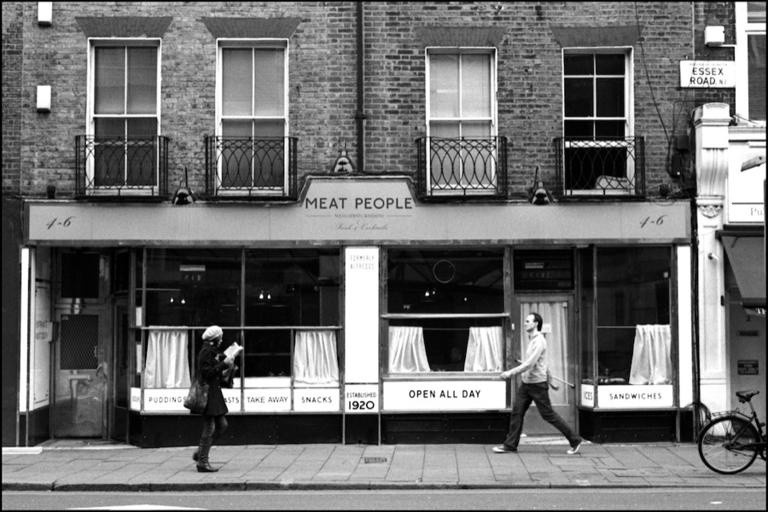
193;437;218;472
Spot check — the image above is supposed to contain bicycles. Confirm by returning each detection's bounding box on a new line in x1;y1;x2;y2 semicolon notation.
698;390;768;475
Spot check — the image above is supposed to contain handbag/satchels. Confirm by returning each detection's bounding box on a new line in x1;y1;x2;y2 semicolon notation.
184;378;209;413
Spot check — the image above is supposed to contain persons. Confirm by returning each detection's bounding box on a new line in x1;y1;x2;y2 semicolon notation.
193;324;234;471
492;313;583;455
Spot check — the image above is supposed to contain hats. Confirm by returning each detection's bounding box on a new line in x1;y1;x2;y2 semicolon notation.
201;325;223;341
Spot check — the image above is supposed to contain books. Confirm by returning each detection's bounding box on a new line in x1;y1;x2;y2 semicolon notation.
222;341;244;361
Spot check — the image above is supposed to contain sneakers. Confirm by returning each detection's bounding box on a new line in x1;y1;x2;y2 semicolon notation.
492;445;519;452
567;438;583;454
